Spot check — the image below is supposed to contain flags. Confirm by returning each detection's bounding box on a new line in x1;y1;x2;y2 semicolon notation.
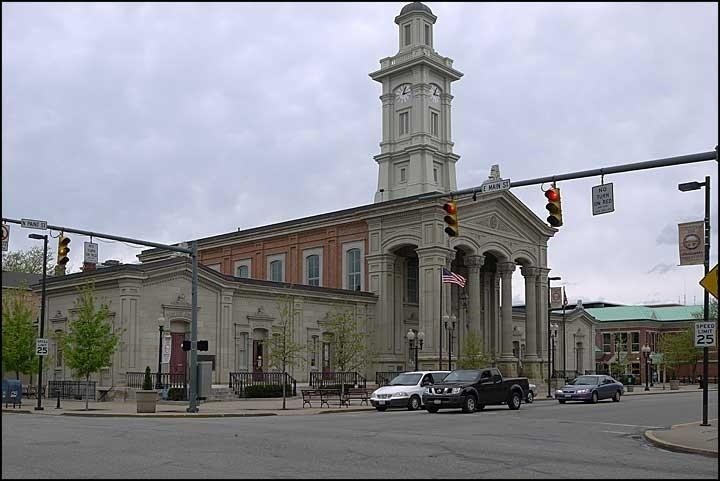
442;267;467;288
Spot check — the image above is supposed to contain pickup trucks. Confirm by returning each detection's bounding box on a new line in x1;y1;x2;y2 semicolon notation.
422;368;529;413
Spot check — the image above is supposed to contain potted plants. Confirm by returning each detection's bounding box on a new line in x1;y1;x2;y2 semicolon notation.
136;365;158;412
669;367;680;390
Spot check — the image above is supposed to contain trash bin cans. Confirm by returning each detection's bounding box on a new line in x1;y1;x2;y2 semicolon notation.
2;379;22;408
627;374;635;384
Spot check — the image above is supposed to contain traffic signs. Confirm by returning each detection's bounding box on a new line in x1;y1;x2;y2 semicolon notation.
481;179;510;195
592;183;614;215
35;338;48;356
695;321;717;347
20;218;48;229
83;241;98;264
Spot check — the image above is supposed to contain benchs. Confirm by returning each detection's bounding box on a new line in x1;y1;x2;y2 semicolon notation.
301;388;376;407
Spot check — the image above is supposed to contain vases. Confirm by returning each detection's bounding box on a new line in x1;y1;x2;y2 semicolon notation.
627;385;633;391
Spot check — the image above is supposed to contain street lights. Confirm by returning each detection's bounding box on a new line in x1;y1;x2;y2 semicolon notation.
550;322;558;379
641;344;655;391
29;233;48;411
443;311;456;370
678;176;710;426
407;328;425;372
155;312;166;389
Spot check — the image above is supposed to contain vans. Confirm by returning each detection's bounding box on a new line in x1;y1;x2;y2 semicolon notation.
370;370;459;411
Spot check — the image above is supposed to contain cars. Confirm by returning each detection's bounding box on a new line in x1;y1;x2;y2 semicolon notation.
555;375;624;404
501;384;538;404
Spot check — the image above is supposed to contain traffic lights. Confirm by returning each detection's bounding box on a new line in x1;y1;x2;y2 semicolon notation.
544;187;563;227
56;235;70;266
443;199;459;237
